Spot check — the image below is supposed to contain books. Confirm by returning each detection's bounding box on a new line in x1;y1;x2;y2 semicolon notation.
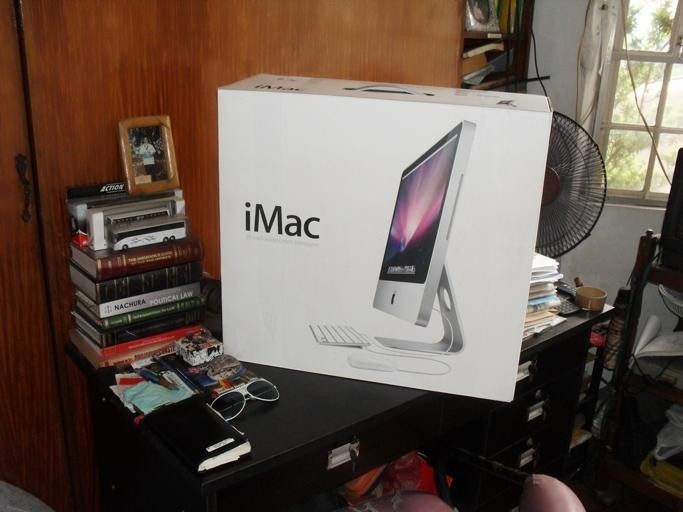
628;314;683;369
66;236;207;370
522;252;567;340
143;392;252;473
152;347;258;402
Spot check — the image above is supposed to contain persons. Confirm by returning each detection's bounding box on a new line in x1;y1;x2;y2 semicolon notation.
139;136;158;182
338;473;587;511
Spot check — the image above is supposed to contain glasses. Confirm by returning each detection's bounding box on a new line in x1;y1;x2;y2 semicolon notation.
211;379;280;421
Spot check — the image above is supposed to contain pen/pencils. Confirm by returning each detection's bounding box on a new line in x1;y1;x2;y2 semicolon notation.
153;354;205;394
140;370;179;390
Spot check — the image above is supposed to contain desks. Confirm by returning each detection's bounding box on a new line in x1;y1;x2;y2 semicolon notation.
59;265;616;512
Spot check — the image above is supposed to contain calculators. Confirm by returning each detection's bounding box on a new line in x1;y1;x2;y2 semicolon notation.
557;294;580;315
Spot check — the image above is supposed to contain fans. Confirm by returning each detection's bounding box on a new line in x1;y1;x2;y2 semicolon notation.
534;111;607;261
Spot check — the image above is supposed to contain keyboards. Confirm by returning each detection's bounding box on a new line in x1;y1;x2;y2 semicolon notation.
310;324;371;349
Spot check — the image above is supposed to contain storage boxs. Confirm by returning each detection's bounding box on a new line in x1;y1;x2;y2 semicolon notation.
218;72;553;406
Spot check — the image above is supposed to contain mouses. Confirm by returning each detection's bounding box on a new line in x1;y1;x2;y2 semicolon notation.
348;351;394;373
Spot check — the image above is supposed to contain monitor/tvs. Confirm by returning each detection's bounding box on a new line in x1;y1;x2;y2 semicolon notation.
374;121;475;355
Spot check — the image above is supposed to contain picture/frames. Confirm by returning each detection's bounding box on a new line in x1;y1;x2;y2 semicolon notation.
463;0;501;35
114;113;183;196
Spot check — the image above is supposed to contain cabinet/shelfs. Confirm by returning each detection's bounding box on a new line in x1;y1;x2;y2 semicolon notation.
602;227;683;511
